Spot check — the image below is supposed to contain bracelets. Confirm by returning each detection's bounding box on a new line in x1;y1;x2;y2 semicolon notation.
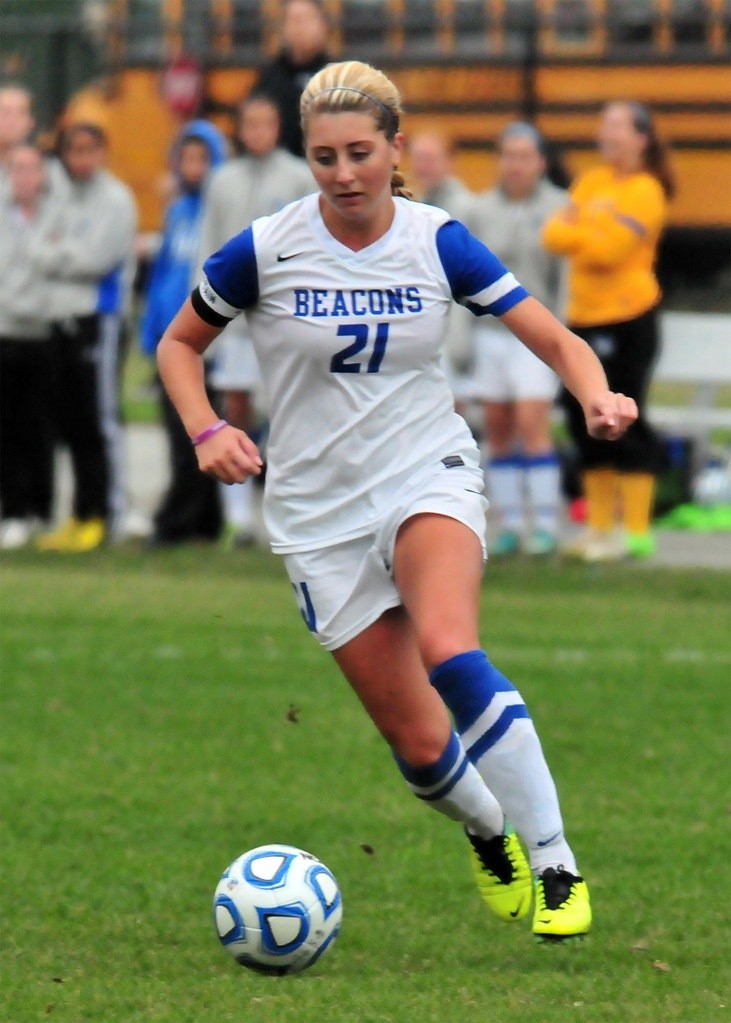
189;420;227;445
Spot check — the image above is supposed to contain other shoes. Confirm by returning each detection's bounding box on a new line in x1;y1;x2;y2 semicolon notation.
565;529;633;563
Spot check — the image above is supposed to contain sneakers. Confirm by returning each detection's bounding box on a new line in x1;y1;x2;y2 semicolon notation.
531;863;593;935
464;815;534;921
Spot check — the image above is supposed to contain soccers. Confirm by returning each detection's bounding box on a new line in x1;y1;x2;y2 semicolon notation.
212;844;344;976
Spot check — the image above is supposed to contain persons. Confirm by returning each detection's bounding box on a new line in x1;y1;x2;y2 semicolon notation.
156;61;638;938
411;101;675;561
0;0;341;551
